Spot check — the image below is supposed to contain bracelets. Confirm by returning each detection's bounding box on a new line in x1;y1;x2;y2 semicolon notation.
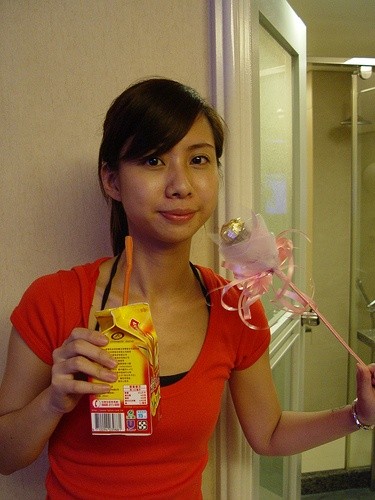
349;396;372;432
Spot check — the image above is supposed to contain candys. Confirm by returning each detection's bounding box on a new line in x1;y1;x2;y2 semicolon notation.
220;219;250;245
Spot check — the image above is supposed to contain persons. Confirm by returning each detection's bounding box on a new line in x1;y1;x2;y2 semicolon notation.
0;75;375;500
